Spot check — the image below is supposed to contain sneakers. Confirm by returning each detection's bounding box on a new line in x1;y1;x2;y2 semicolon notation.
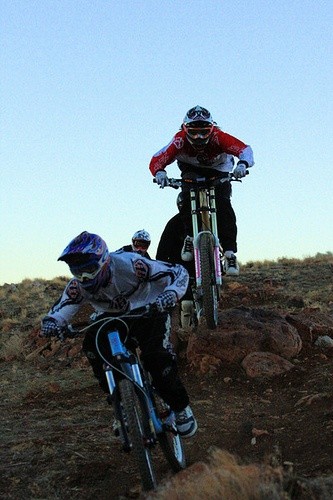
180;235;195;261
224;251;239;276
112;410;128;434
175;404;197;439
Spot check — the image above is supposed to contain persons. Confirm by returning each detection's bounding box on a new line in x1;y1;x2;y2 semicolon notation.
115;229;151;260
149;106;254;277
154;192;199;329
39;231;198;439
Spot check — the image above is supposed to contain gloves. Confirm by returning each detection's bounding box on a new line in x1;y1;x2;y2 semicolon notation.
39;318;64;342
233;162;247;178
156;291;178;313
155;170;170;189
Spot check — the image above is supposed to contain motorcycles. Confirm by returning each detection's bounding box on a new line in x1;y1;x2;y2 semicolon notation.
153;170;249;330
39;301;186;490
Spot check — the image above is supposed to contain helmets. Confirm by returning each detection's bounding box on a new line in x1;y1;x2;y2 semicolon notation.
131;230;151;255
56;231;111;284
181;106;215;144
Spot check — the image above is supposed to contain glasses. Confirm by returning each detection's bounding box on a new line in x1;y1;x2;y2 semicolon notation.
183;125;214;139
133;241;149;248
